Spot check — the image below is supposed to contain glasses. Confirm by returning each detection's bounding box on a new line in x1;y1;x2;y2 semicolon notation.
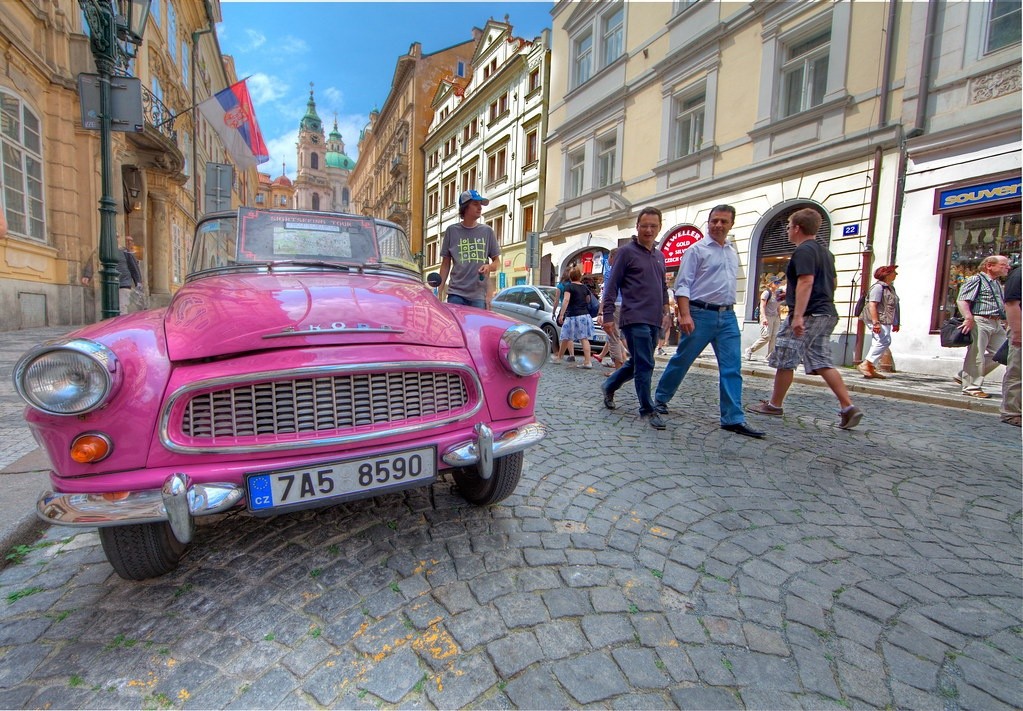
786;225;796;231
637;223;661;231
773;281;781;285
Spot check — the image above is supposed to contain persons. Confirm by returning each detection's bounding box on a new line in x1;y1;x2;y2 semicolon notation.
653;203;766;437
954;254;1012;400
550;267;595;369
600;207;670;430
746;275;782;362
81;230;143;318
1000;259;1022;428
437;189;502;312
551;266;577;362
591;247;678;377
746;207;862;430
857;264;901;380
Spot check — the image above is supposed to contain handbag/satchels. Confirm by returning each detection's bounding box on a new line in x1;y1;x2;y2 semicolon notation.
998;310;1006;320
556;310;567;327
992;338;1008;365
940;316;974;347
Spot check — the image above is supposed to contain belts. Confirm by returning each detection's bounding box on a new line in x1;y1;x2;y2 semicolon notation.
973;314;999;320
689;300;734;312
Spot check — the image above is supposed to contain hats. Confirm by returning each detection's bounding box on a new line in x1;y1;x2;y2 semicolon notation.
874;265;898;280
459;189;489;206
767;276;783;286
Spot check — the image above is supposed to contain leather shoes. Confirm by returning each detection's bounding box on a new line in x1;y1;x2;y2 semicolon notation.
642;410;666;430
654;398;668;414
721;421;767;438
600;383;616;409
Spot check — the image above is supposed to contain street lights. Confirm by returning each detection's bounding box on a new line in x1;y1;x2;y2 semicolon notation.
82;1;157;320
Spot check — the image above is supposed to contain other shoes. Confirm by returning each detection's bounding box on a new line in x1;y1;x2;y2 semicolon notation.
953;376;963;386
962;390;993;398
662;341;669;346
551;350;632;377
1000;415;1022;427
871;371;886;379
856;364;872;379
657;349;667;355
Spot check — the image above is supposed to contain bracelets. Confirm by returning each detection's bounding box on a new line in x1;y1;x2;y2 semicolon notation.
872;320;880;326
663;312;672;320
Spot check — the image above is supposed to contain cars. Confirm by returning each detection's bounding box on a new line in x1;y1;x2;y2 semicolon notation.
13;206;550;578
491;286;610;353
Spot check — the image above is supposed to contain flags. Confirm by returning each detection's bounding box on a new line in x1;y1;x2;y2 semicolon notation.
196;79;269;171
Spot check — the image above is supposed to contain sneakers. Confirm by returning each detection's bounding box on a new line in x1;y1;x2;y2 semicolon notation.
745;399;785;418
745;347;751;360
762;358;769;362
838;405;864;428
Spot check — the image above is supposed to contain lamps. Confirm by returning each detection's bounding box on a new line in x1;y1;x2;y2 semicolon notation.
128;166;140;198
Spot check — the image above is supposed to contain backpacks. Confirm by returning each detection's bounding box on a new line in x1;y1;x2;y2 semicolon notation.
582;284;600;318
855;279;878;317
754;288;772;323
